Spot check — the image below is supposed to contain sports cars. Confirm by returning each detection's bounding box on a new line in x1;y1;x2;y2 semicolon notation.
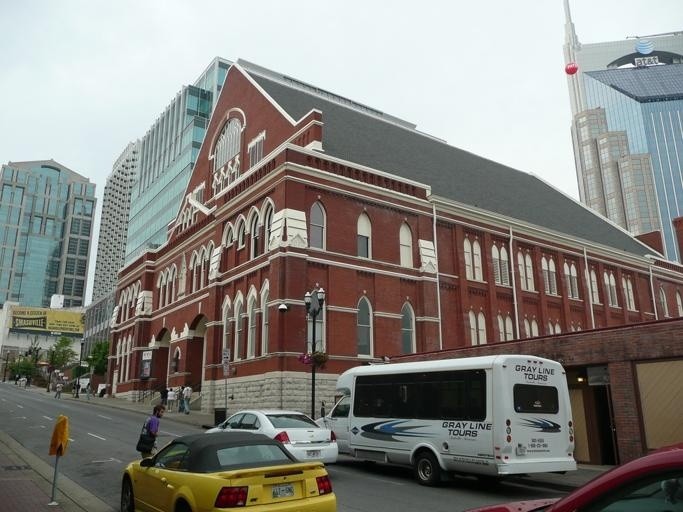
120;429;336;512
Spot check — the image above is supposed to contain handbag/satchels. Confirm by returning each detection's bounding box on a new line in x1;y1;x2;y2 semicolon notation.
136;434;154;453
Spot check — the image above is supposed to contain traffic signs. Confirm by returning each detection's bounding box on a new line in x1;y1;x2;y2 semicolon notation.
223;362;229;375
222;348;230;361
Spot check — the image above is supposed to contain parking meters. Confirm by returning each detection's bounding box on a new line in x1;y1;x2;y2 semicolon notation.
49;414;69;507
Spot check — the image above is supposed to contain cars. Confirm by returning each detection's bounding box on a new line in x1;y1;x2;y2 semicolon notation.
205;408;338;468
460;440;683;512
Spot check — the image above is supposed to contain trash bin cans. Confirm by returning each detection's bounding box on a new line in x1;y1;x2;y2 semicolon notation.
214;407;226;426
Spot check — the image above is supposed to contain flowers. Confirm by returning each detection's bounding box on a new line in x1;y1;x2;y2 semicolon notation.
296;350;312;367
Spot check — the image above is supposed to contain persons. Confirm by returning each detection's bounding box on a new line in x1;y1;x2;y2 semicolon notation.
139;404;165;459
181;383;193;414
177;385;185;413
158;384;168;409
165;387;175;412
13;373;112;400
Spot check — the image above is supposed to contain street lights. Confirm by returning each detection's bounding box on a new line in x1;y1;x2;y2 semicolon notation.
2;351;10;381
44;347;55;392
12;351;22;385
302;285;326;421
74;338;85;398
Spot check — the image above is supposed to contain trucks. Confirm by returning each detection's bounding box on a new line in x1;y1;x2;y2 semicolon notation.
314;353;576;485
